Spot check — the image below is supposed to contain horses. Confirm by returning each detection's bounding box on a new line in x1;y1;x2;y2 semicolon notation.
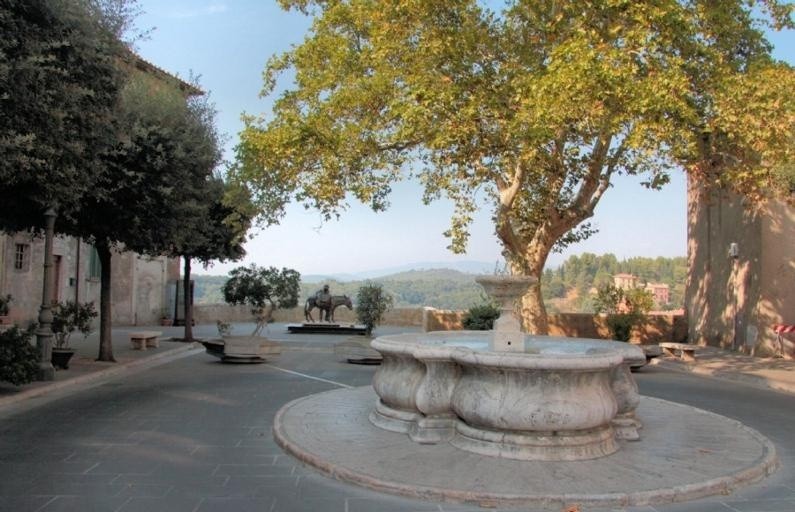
303;295;352;323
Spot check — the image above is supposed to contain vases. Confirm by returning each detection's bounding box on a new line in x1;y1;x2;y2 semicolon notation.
160;319;172;326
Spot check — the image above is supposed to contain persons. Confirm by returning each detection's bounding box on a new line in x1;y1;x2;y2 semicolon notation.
315;284;331;323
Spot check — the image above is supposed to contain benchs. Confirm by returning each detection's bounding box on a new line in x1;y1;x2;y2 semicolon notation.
659;342;697;362
129;331;162;350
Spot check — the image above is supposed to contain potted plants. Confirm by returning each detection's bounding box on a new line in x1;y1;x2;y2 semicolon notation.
50;299;99;370
